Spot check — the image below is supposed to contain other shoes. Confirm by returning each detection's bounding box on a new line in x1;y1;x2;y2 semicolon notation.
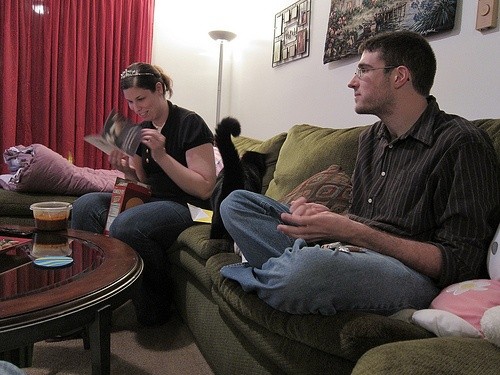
137;292;173;328
45;327;83;342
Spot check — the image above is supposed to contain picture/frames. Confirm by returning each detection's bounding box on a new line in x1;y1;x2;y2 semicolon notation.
271;0;310;67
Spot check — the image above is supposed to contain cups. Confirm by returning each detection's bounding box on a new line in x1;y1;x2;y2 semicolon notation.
29;201;72;231
31;230;69;257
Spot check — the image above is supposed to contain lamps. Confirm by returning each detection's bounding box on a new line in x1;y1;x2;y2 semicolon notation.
209;30;237;132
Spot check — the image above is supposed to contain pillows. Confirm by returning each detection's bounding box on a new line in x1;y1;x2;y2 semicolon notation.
278;164;352;221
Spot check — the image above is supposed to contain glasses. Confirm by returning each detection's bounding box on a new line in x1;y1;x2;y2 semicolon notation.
354;65;410;82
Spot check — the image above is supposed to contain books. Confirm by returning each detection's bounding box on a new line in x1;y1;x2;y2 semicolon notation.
83;108;144;158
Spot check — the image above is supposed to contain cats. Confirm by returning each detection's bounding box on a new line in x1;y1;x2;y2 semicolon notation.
208;116;275;255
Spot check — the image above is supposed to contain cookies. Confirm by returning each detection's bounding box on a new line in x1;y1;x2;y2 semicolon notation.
0;240;19;249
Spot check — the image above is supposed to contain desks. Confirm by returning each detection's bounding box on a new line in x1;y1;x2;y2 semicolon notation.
0;225;144;375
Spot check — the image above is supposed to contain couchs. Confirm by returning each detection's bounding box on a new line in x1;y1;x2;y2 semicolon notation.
0;125;500;375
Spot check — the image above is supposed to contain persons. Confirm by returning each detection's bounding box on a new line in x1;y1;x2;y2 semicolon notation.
46;61;217;341
219;29;500;318
